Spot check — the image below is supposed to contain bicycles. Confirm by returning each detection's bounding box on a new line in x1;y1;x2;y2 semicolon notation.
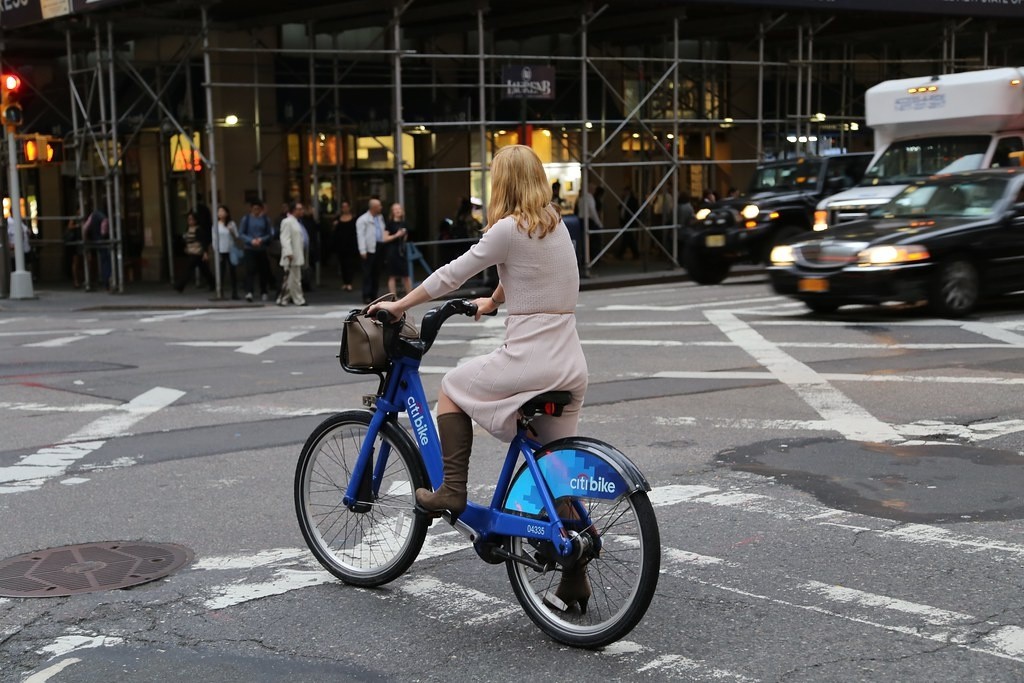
291;296;661;650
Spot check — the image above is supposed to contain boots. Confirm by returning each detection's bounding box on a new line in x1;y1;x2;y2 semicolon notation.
414;412;474;526
543;498;592;614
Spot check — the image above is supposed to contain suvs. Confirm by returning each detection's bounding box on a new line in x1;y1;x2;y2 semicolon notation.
677;148;898;286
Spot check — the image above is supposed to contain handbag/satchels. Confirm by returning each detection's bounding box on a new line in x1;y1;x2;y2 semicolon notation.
346;292;419;368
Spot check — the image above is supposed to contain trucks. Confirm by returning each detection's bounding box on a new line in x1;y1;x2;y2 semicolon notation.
812;64;1024;241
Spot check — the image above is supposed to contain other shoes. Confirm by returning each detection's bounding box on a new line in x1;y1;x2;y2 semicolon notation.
277;301;287;306
260;291;268;301
296;301;308;306
246;291;254;301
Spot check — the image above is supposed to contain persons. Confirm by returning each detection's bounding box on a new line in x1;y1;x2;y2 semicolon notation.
211;205;242;300
356;198;386;305
367;145;591;616
276;203;310;306
301;200;356;292
67;207;112;293
383;203;412;303
170;212;209;290
450;196;483;240
575;190;604;252
550;180;566;210
238;200;274;302
616;189;741;268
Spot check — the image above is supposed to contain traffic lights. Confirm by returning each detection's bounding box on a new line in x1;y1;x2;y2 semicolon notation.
0;73;24;128
18;133;47;161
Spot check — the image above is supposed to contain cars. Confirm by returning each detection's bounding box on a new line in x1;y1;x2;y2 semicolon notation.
764;164;1024;318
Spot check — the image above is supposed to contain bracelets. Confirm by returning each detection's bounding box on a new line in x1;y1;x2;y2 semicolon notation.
490;296;506;305
